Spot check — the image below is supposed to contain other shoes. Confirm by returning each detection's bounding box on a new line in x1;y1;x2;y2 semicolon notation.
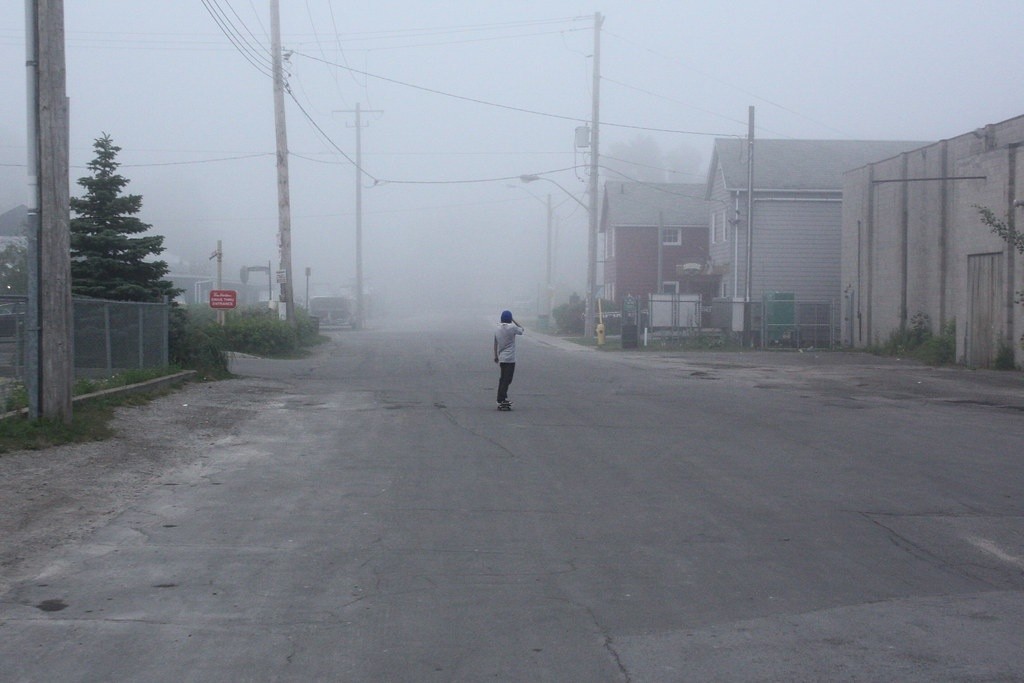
497;400;512;410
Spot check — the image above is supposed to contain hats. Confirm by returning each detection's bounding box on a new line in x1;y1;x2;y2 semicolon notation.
501;310;512;322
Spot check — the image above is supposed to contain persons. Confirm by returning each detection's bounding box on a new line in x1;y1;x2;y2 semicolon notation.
494;310;524;405
